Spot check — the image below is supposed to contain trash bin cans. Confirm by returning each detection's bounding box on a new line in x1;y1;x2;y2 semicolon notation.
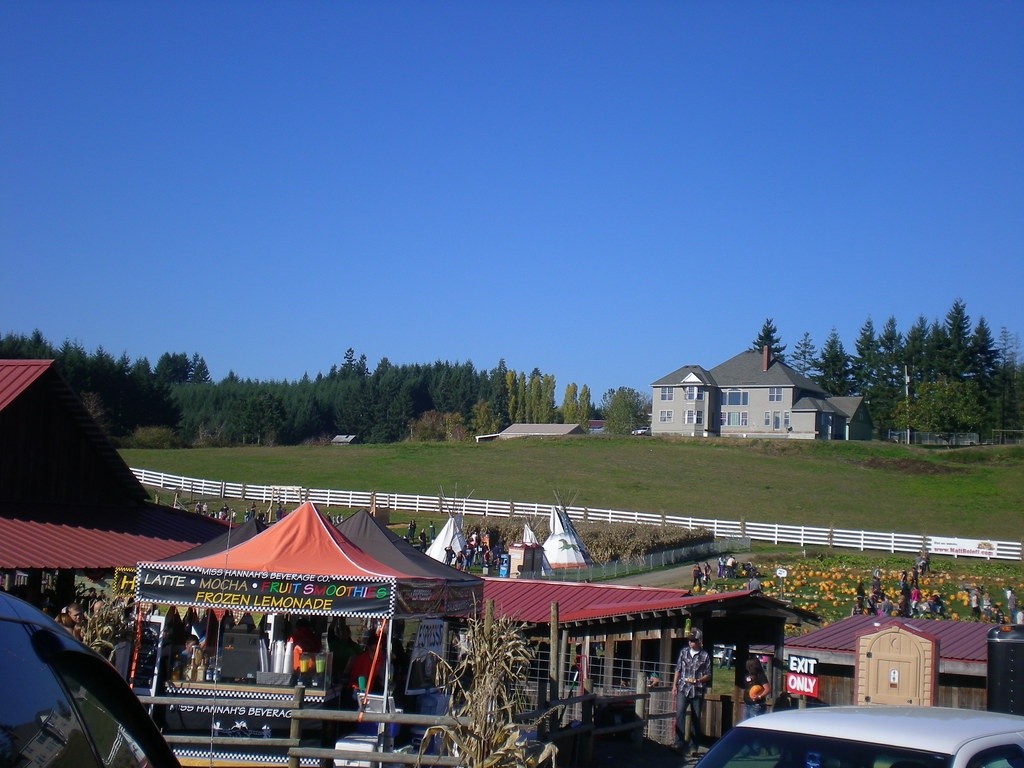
528;543;543;579
508;543;533;579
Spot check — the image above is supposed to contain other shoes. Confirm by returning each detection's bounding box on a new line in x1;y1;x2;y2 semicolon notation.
691;751;697;756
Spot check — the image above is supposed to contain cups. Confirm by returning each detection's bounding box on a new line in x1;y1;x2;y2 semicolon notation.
358;676;366;691
259;639;293;673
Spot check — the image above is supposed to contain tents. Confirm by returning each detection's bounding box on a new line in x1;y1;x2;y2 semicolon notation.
544;506;594;571
422;517;471;566
134;503;485;768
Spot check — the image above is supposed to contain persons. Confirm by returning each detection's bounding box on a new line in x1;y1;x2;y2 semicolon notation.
36;496;1024;768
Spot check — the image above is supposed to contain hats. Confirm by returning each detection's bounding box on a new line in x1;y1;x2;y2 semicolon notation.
61;606;69;613
687;631;700;641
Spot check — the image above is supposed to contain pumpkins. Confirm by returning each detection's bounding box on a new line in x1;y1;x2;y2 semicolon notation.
749;685;763;700
692;564;1024;630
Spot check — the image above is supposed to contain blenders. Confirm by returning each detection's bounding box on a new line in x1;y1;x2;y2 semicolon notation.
297;652;331;689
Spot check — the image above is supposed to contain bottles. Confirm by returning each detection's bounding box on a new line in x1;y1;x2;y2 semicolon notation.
171;646;220;682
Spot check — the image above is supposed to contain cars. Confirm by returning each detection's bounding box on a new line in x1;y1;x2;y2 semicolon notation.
694;706;1024;768
0;592;181;768
